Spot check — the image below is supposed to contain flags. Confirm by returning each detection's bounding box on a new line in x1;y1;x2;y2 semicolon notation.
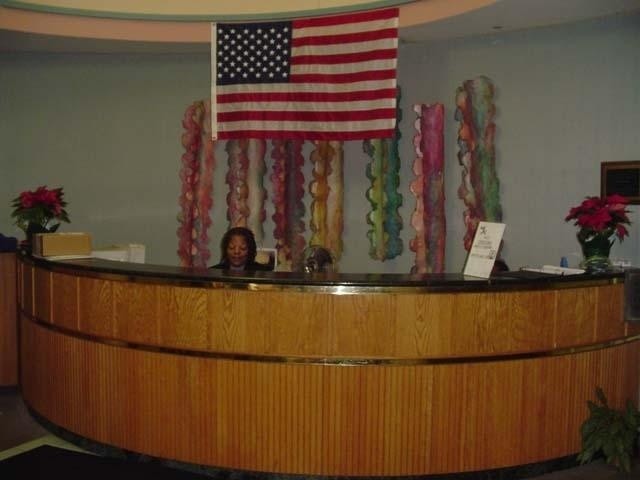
209;2;400;146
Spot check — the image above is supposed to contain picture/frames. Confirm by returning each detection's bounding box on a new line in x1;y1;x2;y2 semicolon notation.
599;159;640;206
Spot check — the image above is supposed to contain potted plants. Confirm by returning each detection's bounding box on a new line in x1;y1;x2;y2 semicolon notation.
580;387;640;475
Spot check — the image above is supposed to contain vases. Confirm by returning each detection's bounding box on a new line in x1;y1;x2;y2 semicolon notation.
581;240;612;263
27;231;53;252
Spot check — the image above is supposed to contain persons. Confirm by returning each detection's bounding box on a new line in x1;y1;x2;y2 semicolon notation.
207;226;273;271
219;268;263;280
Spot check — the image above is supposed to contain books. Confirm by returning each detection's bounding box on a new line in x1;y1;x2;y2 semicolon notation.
521;262;588;275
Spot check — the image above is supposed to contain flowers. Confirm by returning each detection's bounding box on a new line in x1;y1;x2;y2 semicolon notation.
568;195;631;242
10;186;72;233
